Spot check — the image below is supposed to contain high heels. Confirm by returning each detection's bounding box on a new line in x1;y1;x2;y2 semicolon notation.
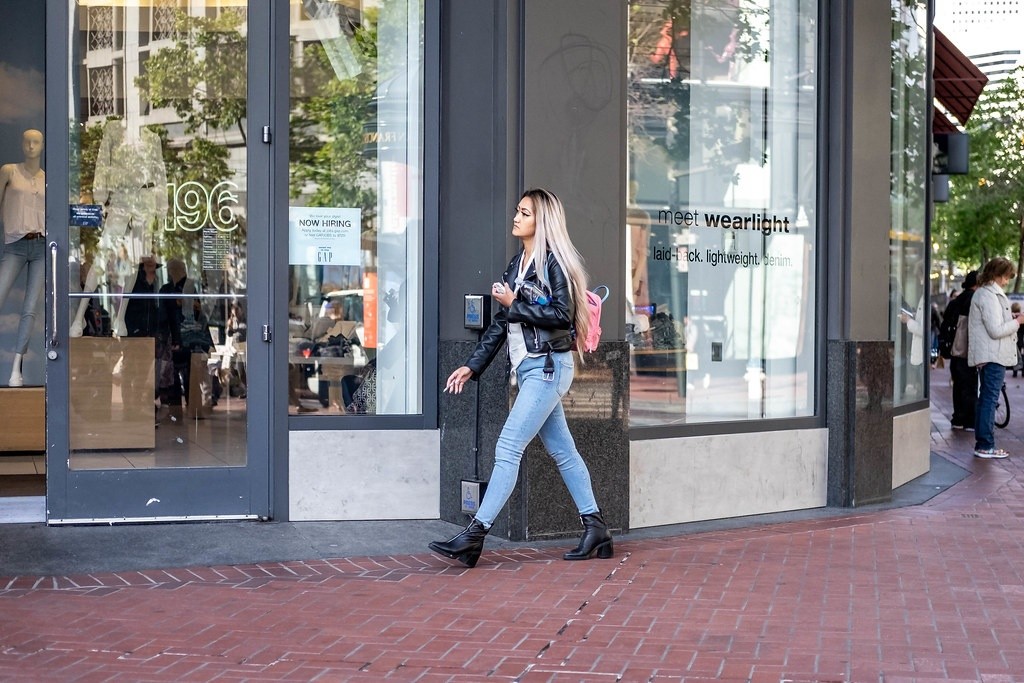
563;508;614;560
427;519;494;568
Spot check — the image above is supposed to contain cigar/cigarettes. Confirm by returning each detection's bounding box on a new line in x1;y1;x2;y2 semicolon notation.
443;382;454;392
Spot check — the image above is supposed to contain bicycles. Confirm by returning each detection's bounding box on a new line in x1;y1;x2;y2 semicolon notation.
977;373;1011;429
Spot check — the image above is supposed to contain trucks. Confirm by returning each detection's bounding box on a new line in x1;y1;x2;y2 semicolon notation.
306;289;378;390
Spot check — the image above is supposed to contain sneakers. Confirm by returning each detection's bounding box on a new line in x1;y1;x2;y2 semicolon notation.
952;421;975;432
974;448;1009;459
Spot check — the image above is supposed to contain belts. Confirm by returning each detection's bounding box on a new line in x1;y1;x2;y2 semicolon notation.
21;232;42;241
141;183;155;189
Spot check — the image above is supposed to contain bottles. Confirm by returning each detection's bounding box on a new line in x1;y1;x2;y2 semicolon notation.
515;276;550;307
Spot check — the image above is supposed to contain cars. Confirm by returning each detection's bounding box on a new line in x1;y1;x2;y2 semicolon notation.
289;320;314;371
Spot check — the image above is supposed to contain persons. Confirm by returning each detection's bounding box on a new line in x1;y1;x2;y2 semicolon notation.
967;258;1024;458
931;270;979;432
429;188;613;567
683;316;698;353
0;130;45;387
68;88;168;338
159;258;216;426
900;266;924;402
124;242;163;427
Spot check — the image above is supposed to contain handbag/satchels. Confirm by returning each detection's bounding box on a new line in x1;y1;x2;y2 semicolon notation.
951;315;968;359
578;284;610;351
